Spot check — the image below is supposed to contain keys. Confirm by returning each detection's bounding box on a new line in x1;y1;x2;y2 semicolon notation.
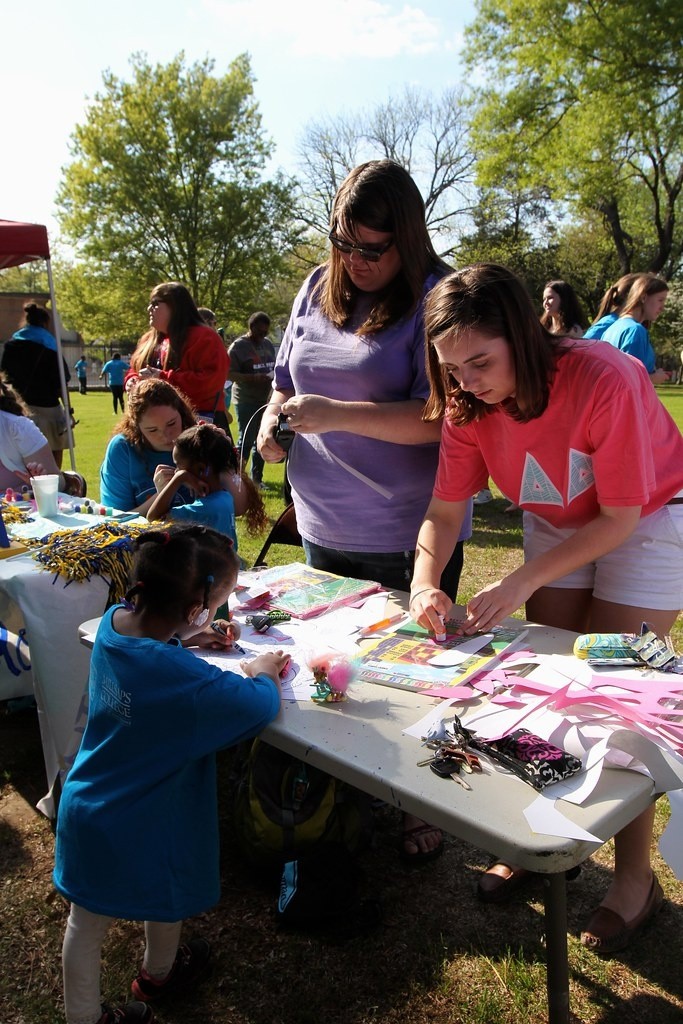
251;615;272;633
416;753;473;790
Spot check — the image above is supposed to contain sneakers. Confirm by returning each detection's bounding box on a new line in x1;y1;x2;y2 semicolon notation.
97;1001;154;1024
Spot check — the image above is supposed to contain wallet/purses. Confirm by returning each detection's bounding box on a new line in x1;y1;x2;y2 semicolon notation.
474;729;582;794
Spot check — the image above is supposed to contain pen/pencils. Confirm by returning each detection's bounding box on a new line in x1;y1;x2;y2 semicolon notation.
362;612;406;634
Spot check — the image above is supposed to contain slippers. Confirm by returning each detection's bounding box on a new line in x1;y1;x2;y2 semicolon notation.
380;807;444;861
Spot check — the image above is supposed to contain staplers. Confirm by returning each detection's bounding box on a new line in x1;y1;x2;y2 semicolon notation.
273;411;295;464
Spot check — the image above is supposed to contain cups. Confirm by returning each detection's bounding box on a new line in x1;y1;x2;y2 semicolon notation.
30;474;59;518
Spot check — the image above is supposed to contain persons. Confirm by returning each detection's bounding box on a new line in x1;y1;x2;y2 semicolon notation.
1;274;672;568
409;265;682;953
249;159;472;606
51;524;292;1024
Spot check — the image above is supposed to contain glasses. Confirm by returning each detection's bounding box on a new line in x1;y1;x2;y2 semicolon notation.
328;225;392;262
149;300;167;308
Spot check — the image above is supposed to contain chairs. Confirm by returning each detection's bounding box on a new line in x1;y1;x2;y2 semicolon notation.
252;503;305;569
60;471;86;497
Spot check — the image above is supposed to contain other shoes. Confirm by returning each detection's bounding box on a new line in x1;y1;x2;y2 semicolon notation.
580;873;664;953
131;938;217;1001
478;859;527;902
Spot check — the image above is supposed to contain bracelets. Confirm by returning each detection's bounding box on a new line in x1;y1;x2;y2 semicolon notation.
410;586;432;608
67;407;74;414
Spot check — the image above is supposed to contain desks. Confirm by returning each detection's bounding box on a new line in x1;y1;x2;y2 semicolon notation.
77;563;683;1024
1;488;151;701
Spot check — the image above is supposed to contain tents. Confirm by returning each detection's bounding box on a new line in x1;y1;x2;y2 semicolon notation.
1;218;81;475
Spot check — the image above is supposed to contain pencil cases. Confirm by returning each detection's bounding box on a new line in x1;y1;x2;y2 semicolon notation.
572;633;637;659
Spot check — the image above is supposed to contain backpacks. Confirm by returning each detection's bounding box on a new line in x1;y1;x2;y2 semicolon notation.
231;737;374;944
